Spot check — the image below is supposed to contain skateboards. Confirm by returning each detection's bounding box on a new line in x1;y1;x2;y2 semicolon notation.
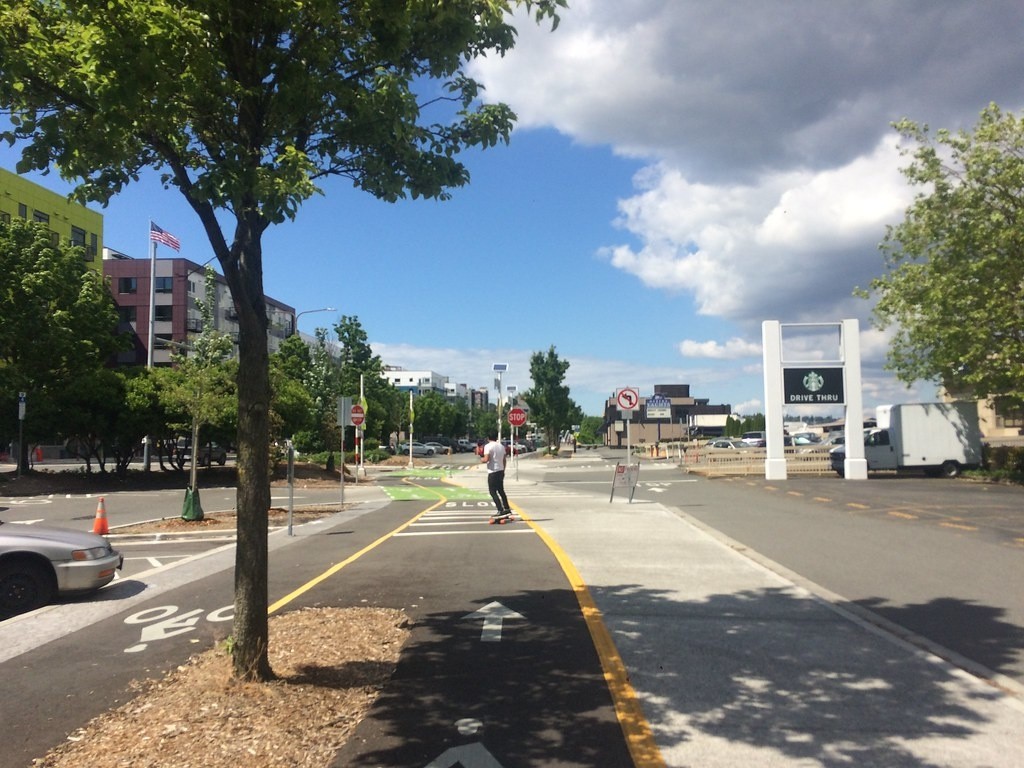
489;511;515;525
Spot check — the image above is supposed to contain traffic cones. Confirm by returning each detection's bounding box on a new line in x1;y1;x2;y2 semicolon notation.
89;497;113;538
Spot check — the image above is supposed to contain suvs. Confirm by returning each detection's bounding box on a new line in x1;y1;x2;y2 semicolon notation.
457;439;478;453
740;430;766;447
401;441;437;456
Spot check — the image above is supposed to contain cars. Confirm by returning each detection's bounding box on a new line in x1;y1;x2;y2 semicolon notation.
0;518;123;614
707;435;732;448
714;439;749;448
476;439;536;458
786;431;844;455
424;442;450;455
171;438;227;466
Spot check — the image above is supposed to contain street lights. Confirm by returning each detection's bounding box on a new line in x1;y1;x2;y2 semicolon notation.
266;306;338;334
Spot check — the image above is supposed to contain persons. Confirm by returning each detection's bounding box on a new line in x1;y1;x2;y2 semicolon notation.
480;428;511;517
387;442;395;455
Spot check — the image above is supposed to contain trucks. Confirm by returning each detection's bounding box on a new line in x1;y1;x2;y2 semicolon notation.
829;399;984;479
783;421;805;435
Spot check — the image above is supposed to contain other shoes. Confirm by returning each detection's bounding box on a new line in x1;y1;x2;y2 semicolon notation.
492;509;512;518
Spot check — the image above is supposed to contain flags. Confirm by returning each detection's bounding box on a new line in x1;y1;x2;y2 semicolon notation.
151;221;180;253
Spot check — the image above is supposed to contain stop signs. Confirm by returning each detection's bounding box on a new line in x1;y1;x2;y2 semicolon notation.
508;408;526;427
350;405;365;426
